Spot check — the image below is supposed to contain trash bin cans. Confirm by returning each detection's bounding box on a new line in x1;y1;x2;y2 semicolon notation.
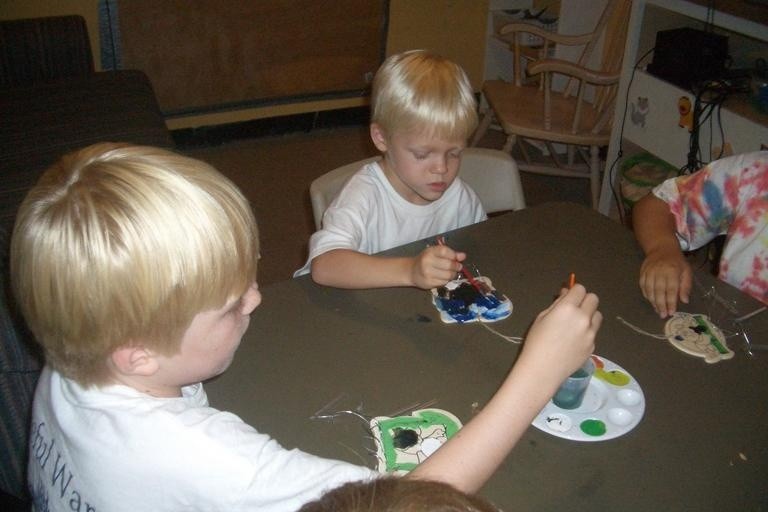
620;155;680;215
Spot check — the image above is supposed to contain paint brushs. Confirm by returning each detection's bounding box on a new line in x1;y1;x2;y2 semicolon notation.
436;236;489;301
569;272;575;290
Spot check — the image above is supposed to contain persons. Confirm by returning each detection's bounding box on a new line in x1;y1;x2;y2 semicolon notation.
1;137;606;511
284;40;492;293
628;149;768;320
296;473;502;509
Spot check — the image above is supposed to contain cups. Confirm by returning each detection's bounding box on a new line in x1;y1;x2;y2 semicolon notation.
552;360;596;410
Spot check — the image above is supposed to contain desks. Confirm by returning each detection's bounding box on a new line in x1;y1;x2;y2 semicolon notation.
203;201;766;511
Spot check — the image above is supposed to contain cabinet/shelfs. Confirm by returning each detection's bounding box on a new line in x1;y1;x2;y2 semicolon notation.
599;0;768;217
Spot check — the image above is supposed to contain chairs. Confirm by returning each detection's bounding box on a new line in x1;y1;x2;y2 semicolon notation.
309;148;526;231
468;1;633;210
